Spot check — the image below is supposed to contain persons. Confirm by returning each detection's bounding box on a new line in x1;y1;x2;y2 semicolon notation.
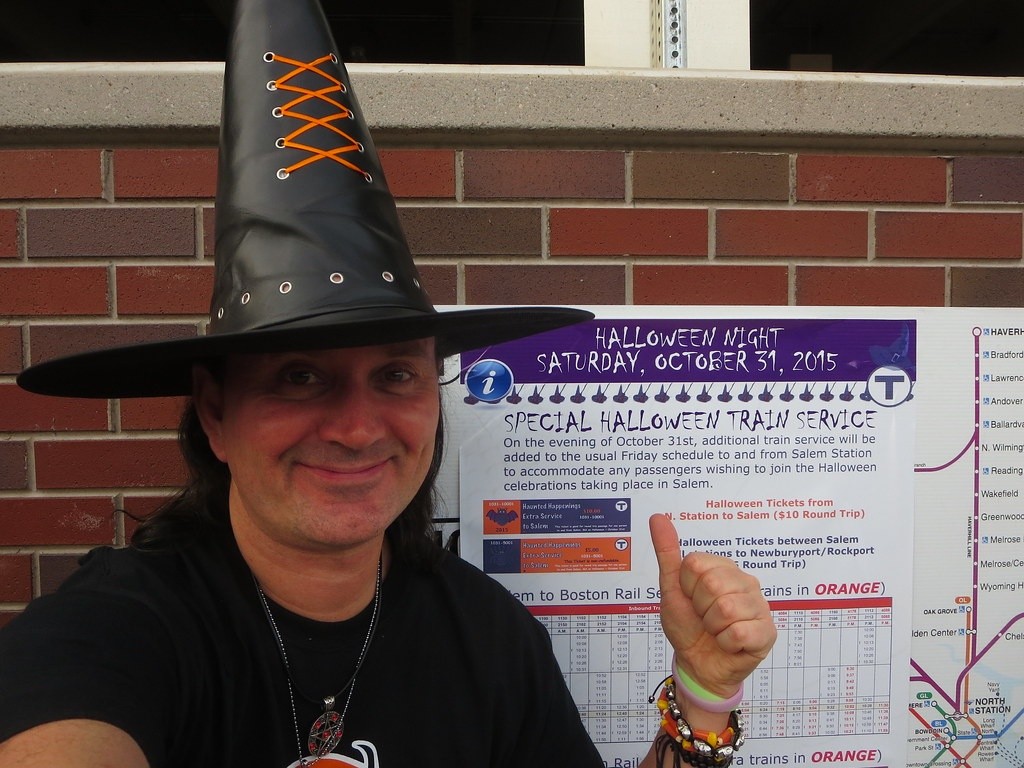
0;328;776;768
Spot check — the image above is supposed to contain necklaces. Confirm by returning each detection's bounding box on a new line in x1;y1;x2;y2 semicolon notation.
252;546;383;768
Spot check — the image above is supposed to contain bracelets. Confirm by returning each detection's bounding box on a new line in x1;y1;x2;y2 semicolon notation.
647;653;745;768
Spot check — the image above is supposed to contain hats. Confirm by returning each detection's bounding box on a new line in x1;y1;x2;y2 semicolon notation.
16;0;595;399
